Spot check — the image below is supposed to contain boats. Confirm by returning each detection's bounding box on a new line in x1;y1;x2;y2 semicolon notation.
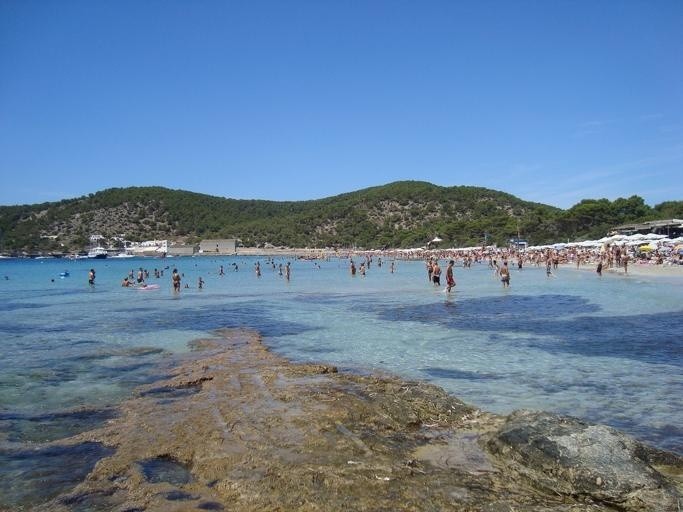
87;246;108;258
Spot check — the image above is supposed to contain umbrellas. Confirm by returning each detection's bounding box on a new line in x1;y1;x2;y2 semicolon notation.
597;232;670;241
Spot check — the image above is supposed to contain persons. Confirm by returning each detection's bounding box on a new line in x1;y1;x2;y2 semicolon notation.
220;251;292;283
350;251;395;277
88;268;95;284
519;242;682;277
122;261;207;294
398;246;523;294
